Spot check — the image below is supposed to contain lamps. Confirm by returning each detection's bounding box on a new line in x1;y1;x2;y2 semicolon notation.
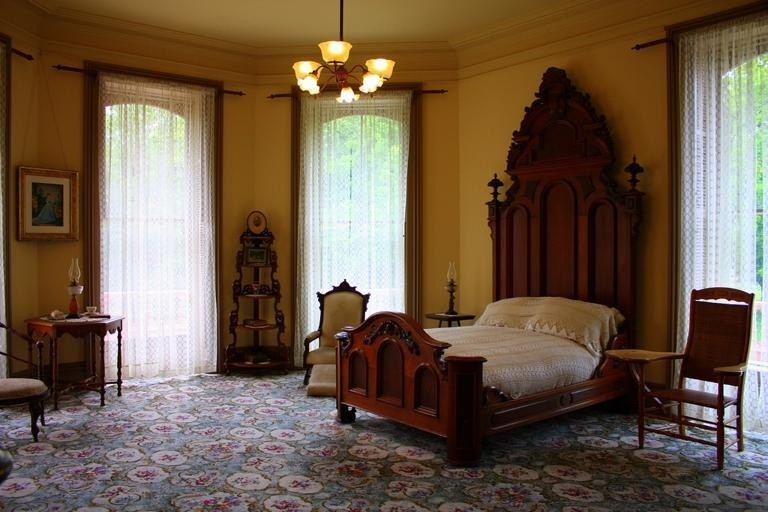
65;256;83;318
291;0;395;102
444;261;459;316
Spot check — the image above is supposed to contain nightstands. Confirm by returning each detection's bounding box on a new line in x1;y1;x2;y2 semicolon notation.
425;314;475;328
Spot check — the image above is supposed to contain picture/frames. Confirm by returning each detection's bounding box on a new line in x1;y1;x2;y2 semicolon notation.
15;165;79;242
246;246;269;264
248;208;267;233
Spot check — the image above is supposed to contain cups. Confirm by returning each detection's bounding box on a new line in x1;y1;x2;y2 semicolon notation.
86;306;97;314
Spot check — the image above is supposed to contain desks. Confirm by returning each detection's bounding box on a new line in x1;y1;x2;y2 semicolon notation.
25;312;127;409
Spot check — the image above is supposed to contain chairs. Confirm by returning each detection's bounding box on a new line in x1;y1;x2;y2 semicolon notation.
302;279;369;398
0;379;50;443
603;287;755;467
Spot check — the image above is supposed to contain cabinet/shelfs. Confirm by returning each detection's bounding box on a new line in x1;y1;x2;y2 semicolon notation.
224;227;292;374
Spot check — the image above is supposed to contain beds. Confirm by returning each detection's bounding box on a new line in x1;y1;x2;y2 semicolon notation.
337;66;649;465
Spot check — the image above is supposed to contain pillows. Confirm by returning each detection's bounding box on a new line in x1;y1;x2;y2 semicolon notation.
475;296;624;358
